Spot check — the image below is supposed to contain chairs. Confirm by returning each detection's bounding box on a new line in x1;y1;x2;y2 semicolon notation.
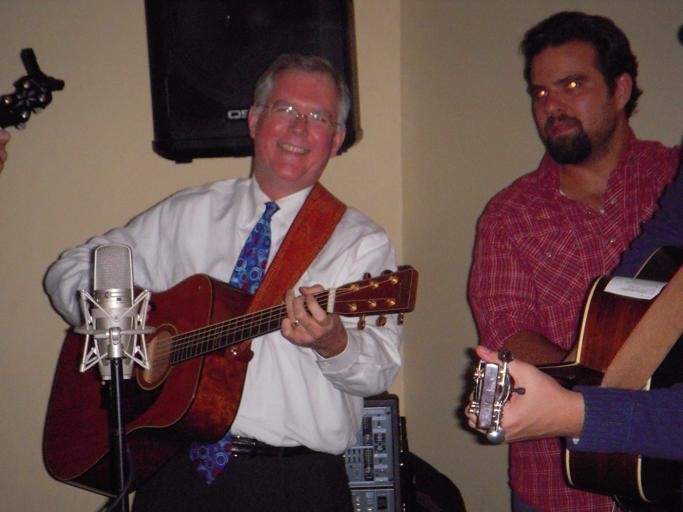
401;452;464;511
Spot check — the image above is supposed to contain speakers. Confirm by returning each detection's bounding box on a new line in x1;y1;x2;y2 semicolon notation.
144;0;364;164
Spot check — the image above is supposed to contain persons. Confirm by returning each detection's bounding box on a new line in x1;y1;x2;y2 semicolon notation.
43;50;406;512
0;124;10;178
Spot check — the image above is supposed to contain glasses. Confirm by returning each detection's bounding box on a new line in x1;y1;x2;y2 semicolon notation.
258;101;342;128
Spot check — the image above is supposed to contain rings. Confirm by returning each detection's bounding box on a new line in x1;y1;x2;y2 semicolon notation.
290;318;300;330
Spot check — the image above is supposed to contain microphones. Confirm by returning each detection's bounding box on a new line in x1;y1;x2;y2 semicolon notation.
92;242;136;381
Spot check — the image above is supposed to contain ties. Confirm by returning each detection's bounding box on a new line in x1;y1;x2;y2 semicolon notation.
187;202;279;490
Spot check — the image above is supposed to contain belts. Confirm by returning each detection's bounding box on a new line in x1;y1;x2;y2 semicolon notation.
231;435;306;457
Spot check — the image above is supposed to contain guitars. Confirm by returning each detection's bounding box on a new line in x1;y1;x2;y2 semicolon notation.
471;245;683;511
42;267;417;500
